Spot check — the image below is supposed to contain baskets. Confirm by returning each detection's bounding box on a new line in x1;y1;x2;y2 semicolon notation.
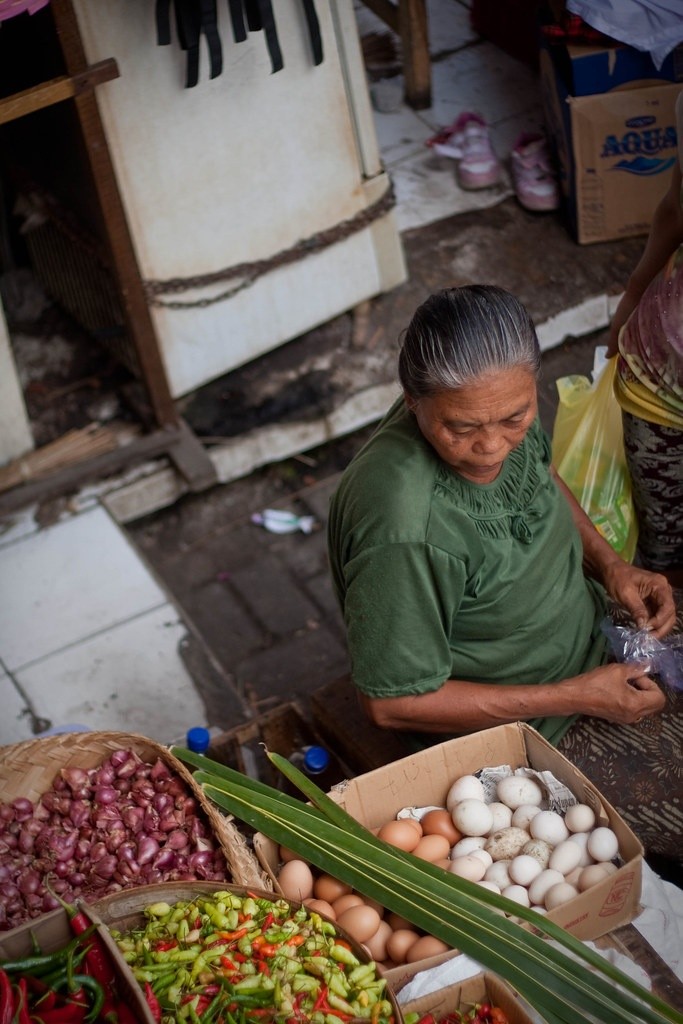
0;732;271;890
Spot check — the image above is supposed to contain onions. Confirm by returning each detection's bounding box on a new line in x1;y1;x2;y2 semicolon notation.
0;747;229;933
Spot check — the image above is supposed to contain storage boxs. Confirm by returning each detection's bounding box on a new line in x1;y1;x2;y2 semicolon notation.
397;972;549;1024
535;38;683;245
251;721;643;994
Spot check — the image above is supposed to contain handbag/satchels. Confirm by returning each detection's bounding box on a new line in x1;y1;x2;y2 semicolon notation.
548;352;637;568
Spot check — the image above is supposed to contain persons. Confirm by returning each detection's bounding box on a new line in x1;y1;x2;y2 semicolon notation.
605;86;683;592
327;284;683;882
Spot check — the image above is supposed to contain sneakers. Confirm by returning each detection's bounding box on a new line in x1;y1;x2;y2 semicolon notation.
508;131;557;209
425;112;502;190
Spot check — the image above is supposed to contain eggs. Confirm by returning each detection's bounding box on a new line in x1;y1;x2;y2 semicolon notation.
278;776;620;971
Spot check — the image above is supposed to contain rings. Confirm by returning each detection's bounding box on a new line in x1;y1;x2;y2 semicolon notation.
635;717;641;723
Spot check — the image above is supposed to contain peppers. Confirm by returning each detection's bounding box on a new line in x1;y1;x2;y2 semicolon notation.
0;873;144;1024
109;888;512;1024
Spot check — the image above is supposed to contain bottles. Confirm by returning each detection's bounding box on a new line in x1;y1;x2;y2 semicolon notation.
277;744;335;800
177;728;231;791
